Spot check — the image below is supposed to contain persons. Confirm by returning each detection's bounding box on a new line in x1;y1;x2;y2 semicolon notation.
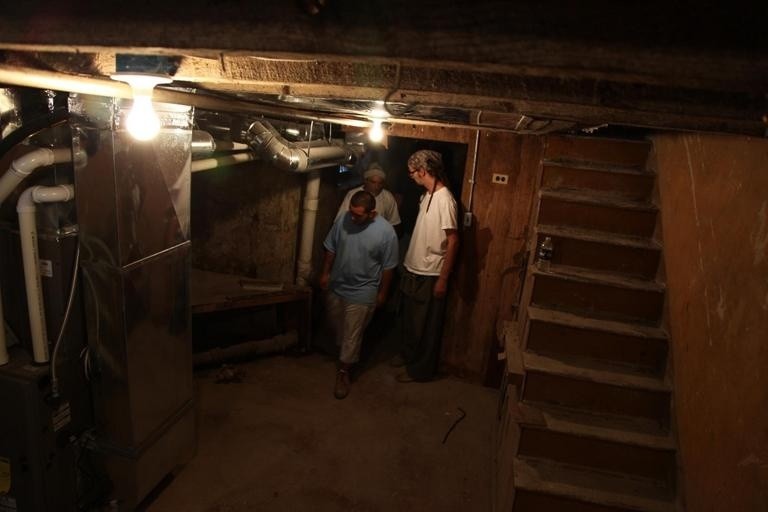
332;162;402;364
390;150;459;383
319;189;401;399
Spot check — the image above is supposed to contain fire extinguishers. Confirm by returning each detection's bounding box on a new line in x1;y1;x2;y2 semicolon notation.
64;430;106;511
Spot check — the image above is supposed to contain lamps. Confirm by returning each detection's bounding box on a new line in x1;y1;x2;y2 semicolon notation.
110;71;173;142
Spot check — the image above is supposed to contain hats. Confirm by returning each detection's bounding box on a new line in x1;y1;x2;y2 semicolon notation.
363;162;386;180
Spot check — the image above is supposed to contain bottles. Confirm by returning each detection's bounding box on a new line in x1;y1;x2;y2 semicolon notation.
537;235;553;273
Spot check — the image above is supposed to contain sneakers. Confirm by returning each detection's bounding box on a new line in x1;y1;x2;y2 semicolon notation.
391;353;433;382
334;369;352;399
